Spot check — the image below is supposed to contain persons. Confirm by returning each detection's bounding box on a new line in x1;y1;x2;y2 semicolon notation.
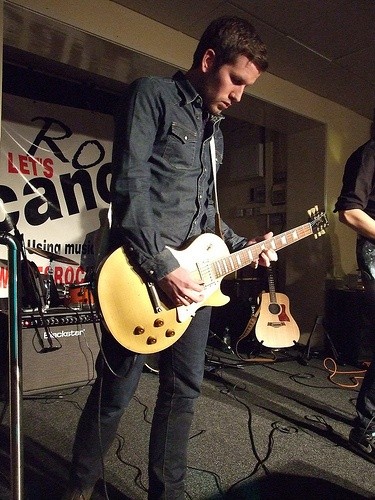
333;138;375;463
64;18;277;500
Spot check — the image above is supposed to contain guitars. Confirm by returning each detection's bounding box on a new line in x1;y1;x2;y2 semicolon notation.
94;202;331;356
254;262;300;349
361;239;375;280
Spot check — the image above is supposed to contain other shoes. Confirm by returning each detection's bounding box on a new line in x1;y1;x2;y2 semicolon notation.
348;428;375;464
63;484;94;500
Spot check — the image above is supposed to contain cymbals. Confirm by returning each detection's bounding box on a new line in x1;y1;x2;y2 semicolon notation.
25;247;79;266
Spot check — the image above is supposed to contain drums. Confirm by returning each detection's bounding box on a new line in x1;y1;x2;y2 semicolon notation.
63;282;94;308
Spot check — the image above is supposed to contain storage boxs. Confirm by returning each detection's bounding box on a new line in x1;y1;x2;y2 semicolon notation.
0;309;103;397
